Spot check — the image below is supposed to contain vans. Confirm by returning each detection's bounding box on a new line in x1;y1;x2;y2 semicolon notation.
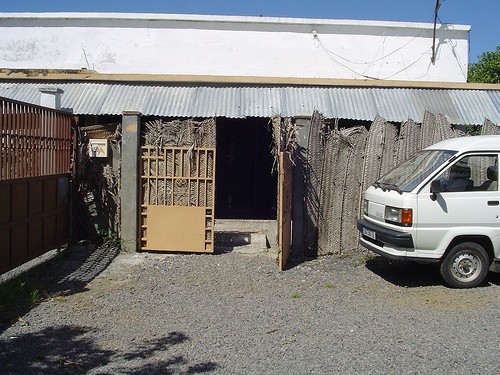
359;134;500;289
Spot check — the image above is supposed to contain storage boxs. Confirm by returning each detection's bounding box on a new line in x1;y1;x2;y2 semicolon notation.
89;138;108;158
